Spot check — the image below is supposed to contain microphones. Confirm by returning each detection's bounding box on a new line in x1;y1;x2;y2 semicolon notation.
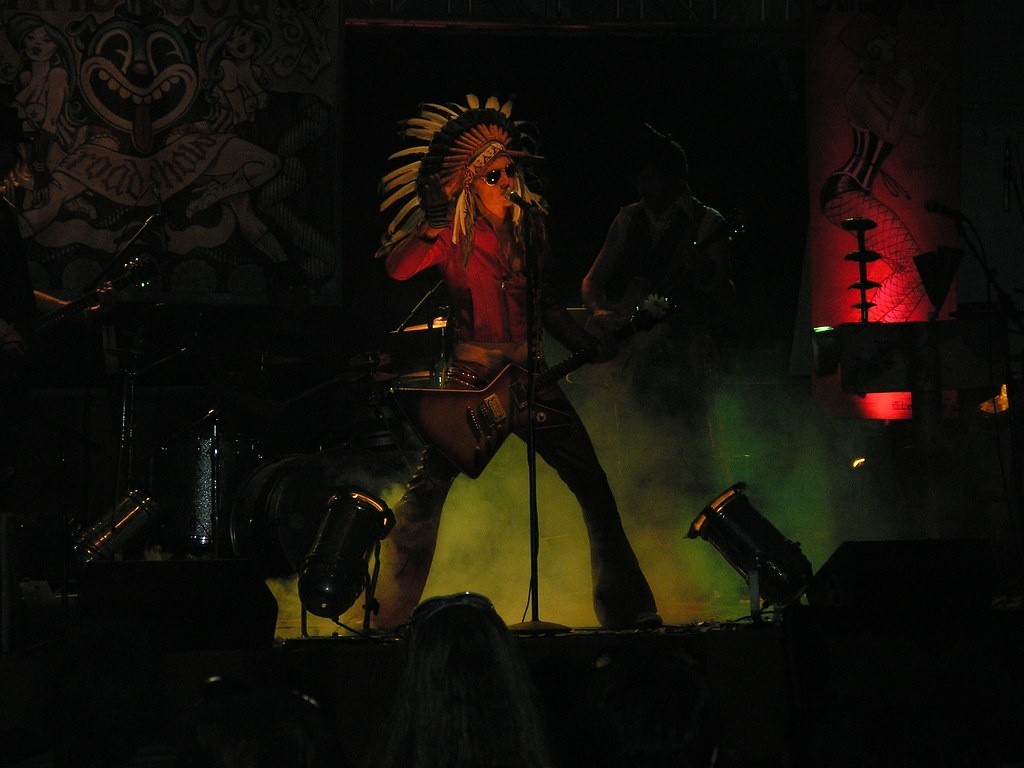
154;184;166;240
924;199;970;224
508;191;536;215
1003;146;1010;211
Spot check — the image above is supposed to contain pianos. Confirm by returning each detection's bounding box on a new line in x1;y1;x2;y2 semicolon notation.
818;316;1024;394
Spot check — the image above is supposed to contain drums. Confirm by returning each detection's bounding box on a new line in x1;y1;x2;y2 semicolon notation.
189;435;223;550
349;369;439;451
225;451;346;580
76;488;158;567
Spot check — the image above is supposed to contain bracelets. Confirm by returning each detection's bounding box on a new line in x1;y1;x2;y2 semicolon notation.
415;220;439;245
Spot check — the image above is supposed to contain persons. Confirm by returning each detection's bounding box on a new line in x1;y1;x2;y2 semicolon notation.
354;92;661;637
579;142;731;521
178;669;338;768
366;592;548;767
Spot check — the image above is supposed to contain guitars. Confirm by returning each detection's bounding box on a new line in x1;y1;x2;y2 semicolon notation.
655;206;746;303
0;257;159;383
386;293;673;479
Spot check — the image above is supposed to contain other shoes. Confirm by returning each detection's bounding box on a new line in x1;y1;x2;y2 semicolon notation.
629;610;661;630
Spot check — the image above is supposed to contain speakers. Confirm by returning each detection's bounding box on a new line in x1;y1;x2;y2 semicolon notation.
82;560;278;652
805;539;994;616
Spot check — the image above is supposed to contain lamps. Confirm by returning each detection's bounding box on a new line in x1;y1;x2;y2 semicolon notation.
690;487;812;609
296;486;396;638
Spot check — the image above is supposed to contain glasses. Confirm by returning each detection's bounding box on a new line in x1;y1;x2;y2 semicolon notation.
475;162;520;187
